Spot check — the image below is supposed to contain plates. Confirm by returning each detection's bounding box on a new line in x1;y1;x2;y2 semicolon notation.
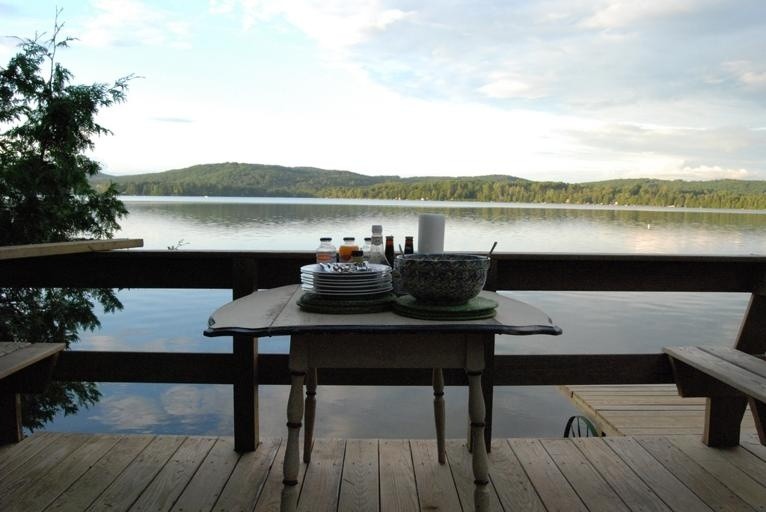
296;263;398;314
395;294;499;320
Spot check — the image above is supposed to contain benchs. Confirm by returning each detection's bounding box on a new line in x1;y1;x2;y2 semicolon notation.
658;345;765;450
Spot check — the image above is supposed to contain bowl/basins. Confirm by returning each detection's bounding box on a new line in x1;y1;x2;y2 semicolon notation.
397;252;492;306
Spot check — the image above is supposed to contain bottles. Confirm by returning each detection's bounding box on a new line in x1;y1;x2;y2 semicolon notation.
314;237;338;264
368;224;391;266
339;238;359;263
363;238;372;261
405;236;414;255
349;251;365;262
385;235;395;266
